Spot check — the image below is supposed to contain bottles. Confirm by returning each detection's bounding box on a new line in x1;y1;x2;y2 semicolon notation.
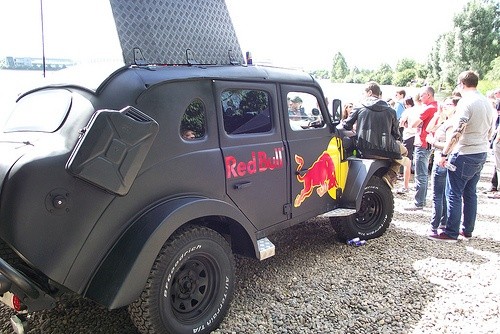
440;161;457;172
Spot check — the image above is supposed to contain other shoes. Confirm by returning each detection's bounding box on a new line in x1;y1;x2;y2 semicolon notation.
459;229;472;237
397;187;408;194
439;224;445;228
404;204;422;210
483;187;496;194
487;192;500;199
430;232;457;240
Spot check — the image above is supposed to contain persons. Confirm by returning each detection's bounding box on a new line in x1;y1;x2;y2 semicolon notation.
404;87;439;211
427;70;495;241
288;96;357;132
395;90;406;119
425;96;461;238
342;81;408;190
385;98;395;108
485;89;500;198
397;93;425;194
406;97;415;109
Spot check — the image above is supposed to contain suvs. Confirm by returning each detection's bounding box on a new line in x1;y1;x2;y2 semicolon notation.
0;61;396;334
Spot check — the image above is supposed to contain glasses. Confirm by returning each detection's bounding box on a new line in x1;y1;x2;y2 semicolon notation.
455;82;462;89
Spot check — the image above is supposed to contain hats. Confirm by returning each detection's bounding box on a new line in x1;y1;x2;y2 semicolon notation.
291;97;302;103
